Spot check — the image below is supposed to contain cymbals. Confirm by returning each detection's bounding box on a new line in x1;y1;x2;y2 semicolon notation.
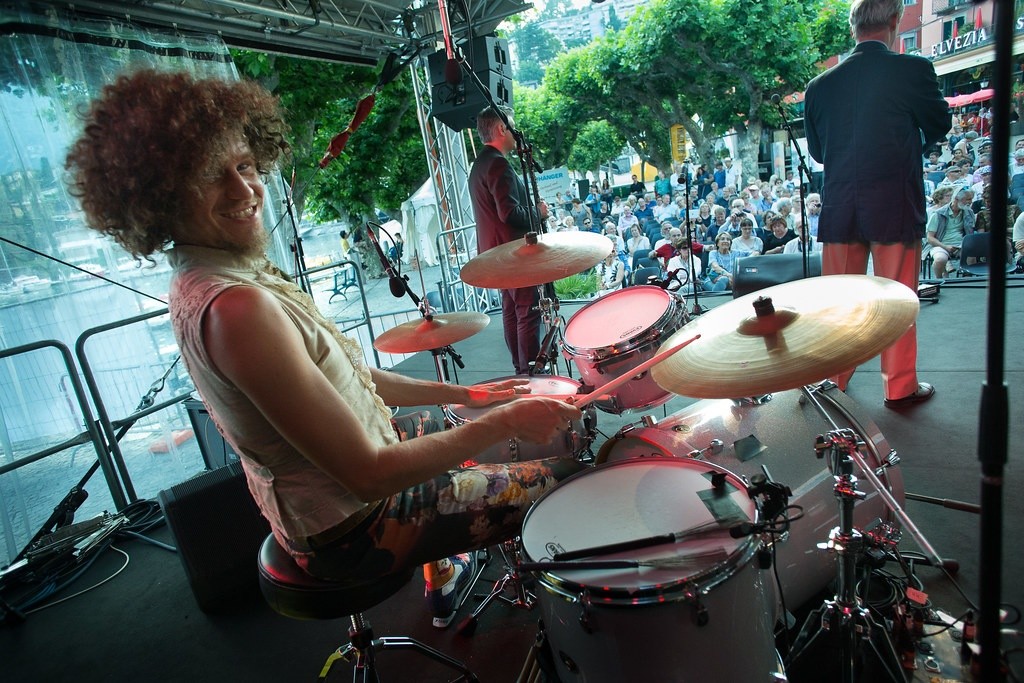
374;309;491;354
458;228;614;290
649;273;921;400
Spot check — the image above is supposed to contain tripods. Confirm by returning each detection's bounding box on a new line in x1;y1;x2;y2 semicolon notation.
686;171;710;316
784;384;945;683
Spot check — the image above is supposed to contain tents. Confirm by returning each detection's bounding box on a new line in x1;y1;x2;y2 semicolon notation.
400;162;476;267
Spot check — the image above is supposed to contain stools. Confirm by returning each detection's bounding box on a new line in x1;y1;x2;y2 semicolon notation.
260;530;477;683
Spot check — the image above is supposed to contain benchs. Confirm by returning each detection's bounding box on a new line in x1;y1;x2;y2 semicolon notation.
323;265;358;304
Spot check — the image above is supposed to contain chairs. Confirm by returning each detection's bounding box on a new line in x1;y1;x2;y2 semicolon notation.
606;171;1024;288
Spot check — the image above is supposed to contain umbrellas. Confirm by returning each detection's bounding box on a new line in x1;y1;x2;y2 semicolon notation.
942;95;958;119
972;88;994;138
951;93;973;123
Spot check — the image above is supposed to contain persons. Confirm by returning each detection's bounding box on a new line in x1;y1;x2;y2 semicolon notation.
467;104;553;376
66;70;582;628
543;93;1024;299
803;0;953;408
339;229;404;281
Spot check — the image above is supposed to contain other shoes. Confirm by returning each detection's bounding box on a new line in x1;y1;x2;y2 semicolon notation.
424;553;475;619
884;382;935;408
515;366;551;376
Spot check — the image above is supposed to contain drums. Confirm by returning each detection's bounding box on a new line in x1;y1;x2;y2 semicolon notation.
520;459;784;679
443;373;592;470
594;378;908;634
565;282;689;415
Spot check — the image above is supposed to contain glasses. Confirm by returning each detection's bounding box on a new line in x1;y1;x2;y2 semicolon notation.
681;247;689;250
951;170;962;173
584;222;590;225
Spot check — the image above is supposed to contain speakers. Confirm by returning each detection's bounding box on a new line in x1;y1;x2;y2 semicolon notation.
427;36;512;133
733;250;822;300
157;459;271;616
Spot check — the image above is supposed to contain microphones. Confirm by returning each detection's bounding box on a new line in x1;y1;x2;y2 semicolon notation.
367;225;407;297
437;0;462;84
535;315;563;368
660;268;680;289
730;521;753;539
677;164;687;184
772;94;784;113
